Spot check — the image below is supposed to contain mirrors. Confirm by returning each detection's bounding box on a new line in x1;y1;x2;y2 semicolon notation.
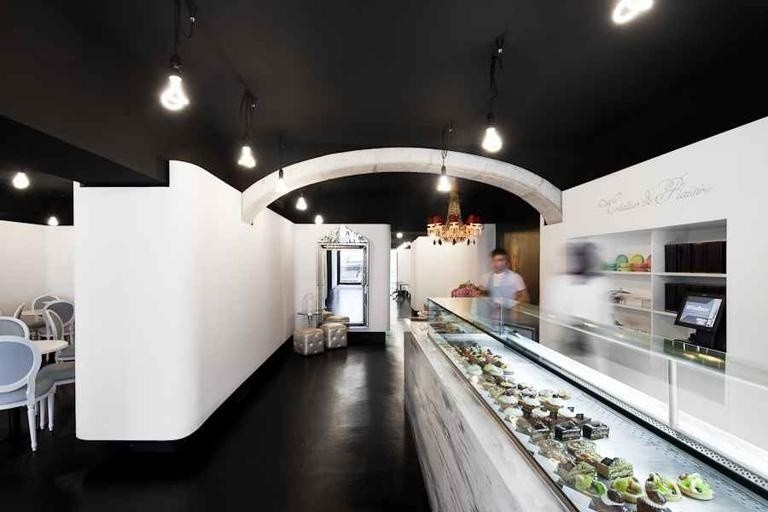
315;224;370;326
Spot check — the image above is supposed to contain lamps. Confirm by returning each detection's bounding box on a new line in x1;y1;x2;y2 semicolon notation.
432;118;464;193
426;174;485;248
478;35;517;153
293;146;328;225
156;0;200;112
234;71;262;169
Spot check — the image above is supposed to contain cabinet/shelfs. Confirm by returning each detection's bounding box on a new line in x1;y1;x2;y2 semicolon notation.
562;215;731;413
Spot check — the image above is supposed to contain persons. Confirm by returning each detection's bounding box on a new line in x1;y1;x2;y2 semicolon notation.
478;247;531;323
554;240;616;366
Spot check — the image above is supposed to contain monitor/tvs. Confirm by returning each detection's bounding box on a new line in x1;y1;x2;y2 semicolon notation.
673;291;726;347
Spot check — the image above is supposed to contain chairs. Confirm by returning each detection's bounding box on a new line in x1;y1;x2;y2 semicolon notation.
0;292;75;451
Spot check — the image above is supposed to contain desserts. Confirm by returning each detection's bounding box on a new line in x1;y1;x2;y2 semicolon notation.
462;343;715;510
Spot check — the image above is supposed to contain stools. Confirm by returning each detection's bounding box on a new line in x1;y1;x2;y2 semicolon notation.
292;309;349;357
